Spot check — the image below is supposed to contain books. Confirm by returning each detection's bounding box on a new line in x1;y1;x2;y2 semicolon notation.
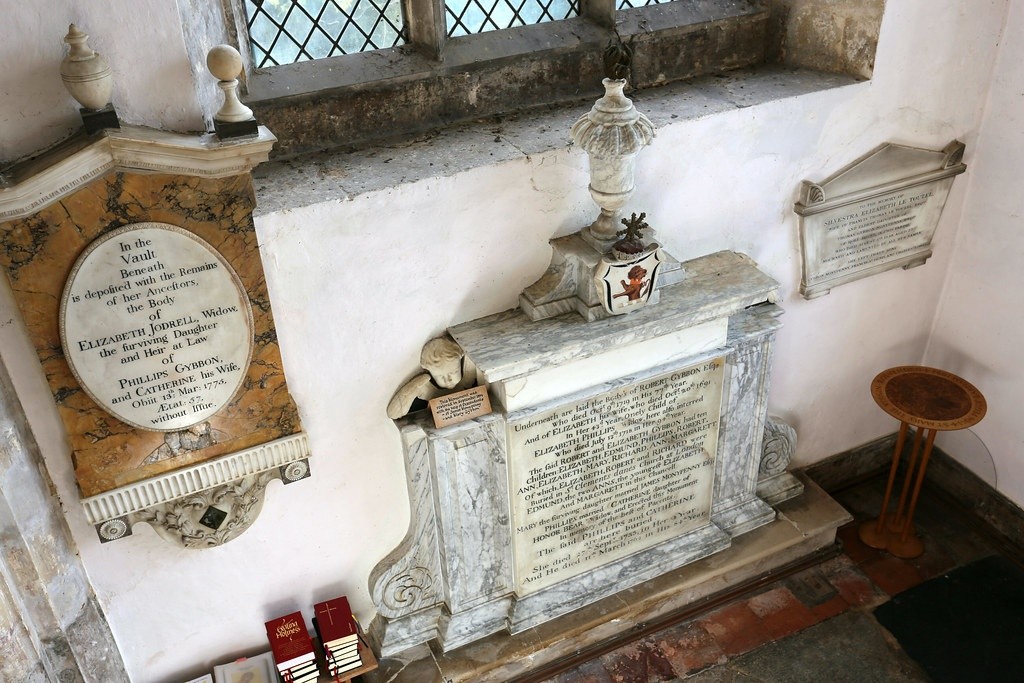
312;595;362;678
265;610;321;683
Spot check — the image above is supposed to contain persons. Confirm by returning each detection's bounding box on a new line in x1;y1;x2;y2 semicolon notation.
421;337;464;389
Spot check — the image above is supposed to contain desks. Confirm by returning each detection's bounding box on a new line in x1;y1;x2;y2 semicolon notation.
859;368;988;558
